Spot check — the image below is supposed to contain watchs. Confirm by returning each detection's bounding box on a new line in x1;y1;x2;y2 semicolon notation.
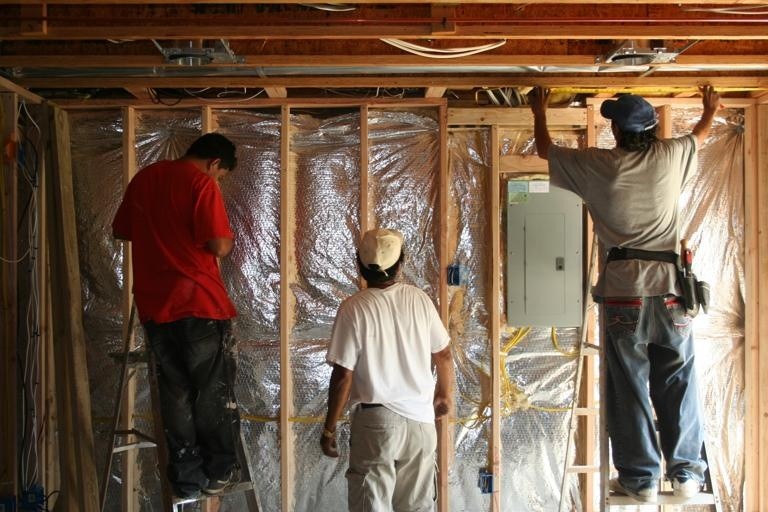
324;429;336;438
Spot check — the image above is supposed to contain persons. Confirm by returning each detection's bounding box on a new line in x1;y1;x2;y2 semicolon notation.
528;84;719;504
113;132;242;500
320;229;454;512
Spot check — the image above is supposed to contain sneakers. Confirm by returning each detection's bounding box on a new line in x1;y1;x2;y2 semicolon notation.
673;475;701;498
201;462;242;495
609;478;659;503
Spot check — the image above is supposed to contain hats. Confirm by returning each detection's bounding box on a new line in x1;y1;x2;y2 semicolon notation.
600;96;657;132
359;228;404;273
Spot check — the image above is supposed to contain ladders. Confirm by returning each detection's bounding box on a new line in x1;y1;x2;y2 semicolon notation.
100;293;263;512
559;230;723;512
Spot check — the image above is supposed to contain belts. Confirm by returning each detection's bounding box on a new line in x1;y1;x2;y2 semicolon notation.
361;402;380;409
